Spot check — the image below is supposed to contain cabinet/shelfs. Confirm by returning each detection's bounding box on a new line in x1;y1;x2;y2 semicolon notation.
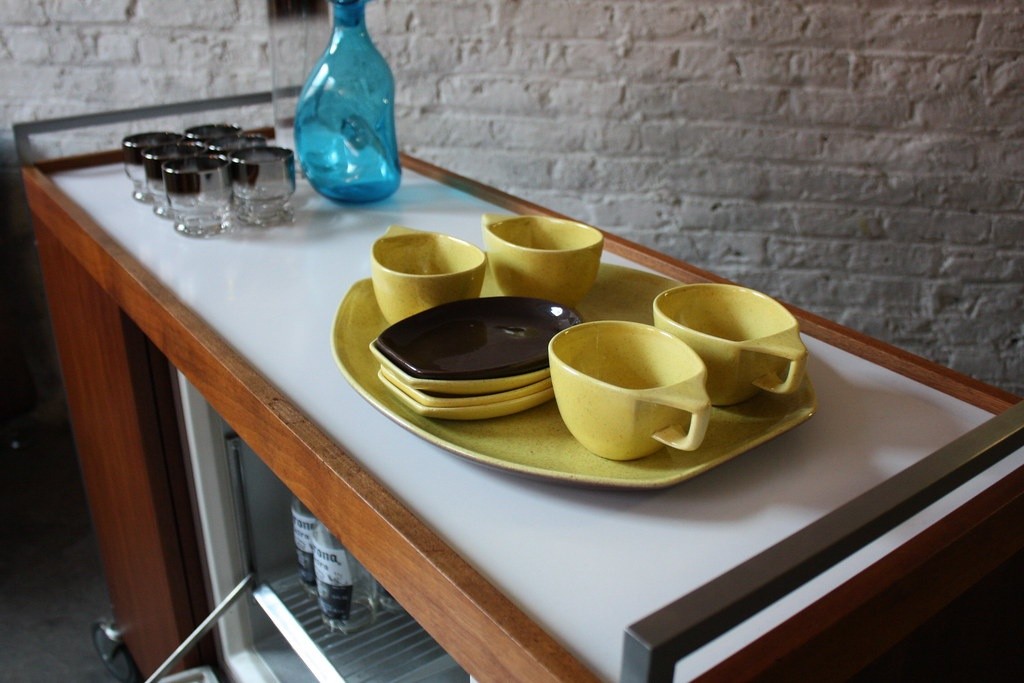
20;119;1024;682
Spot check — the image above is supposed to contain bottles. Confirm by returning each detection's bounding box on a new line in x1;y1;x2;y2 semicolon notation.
293;0;403;202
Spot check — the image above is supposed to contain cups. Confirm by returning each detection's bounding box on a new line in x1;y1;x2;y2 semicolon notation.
370;224;486;325
290;490;406;633
121;123;298;238
480;211;604;309
548;320;711;461
652;283;808;406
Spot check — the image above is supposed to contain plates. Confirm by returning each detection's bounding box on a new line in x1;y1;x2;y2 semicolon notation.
370;293;583;418
